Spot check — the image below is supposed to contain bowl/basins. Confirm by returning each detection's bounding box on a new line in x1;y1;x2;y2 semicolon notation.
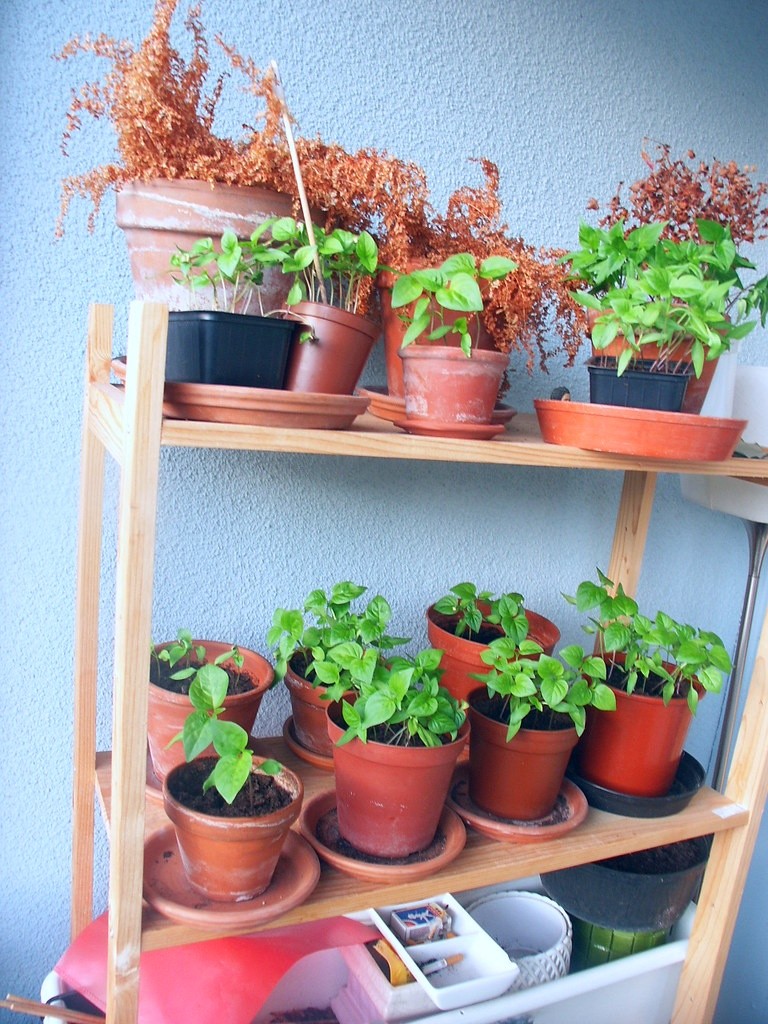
540;835;710;933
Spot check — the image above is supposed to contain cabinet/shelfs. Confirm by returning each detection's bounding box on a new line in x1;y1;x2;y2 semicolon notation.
73;304;768;1024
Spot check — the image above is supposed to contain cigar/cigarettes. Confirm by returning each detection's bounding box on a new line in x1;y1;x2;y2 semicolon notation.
420;953;464;976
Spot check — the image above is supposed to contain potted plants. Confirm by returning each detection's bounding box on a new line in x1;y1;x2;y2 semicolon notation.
49;0;424;308
581;139;768;409
251;215;389;392
162;663;305;902
273;582;415;753
309;642;473;858
567;565;731;800
361;157;591;377
145;636;274;777
389;251;523;420
556;218;768;412
426;580;564;704
161;230;310;382
463;636;617;817
